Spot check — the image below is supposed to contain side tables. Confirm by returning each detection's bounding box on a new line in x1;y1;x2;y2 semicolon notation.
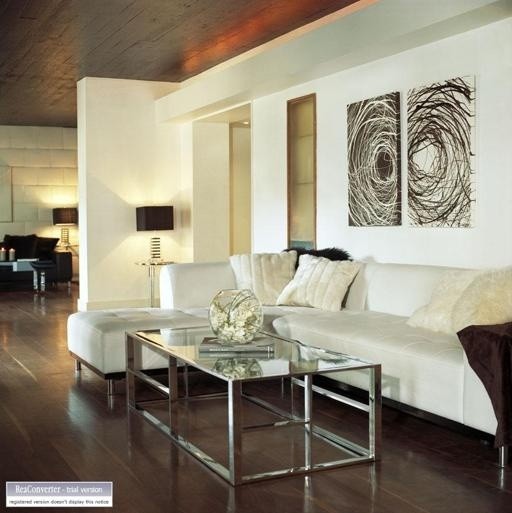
135;261;176;307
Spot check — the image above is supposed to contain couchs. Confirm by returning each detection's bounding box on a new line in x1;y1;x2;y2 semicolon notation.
66;261;512;470
1;232;58;264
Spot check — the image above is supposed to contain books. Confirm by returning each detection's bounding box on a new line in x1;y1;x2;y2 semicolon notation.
197;335;277;354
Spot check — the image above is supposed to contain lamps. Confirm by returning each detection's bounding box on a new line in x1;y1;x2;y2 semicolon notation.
137;206;175;263
53;207;80;246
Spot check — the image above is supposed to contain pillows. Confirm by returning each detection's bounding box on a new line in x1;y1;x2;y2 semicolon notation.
227;250;297;306
276;254;362;312
405;265;512;335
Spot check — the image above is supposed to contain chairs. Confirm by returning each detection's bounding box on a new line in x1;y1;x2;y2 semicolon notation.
31;252;73;298
